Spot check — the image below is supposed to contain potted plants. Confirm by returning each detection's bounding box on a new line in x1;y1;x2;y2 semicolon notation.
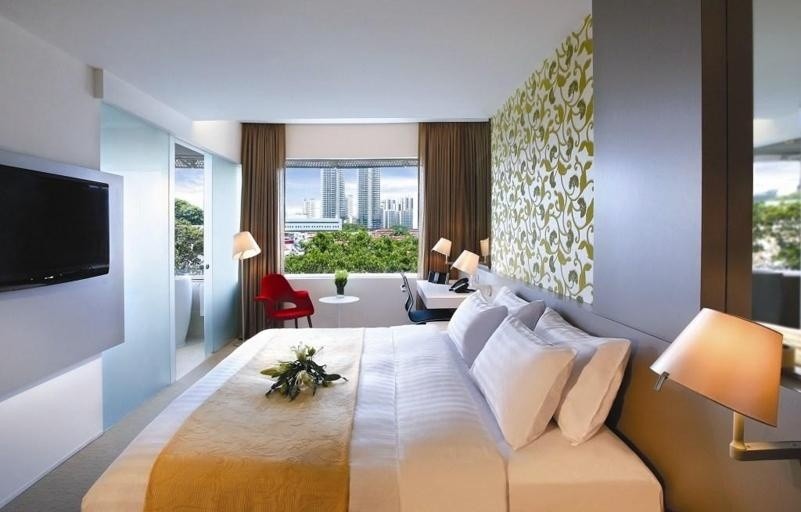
334;269;349;299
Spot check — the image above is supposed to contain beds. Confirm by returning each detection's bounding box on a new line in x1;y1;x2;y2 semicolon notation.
81;327;665;512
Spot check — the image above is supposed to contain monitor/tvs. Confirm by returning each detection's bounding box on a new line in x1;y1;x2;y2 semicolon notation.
0;163;111;293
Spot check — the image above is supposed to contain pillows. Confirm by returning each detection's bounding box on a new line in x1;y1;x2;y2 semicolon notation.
494;286;545;331
468;313;578;449
534;306;632;448
447;290;508;370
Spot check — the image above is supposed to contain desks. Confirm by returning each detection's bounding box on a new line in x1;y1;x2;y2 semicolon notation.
416;279;476;310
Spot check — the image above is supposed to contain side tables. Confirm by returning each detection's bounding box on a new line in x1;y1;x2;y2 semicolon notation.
319;296;360;328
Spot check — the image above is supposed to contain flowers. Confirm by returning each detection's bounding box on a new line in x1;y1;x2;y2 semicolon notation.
259;339;349;403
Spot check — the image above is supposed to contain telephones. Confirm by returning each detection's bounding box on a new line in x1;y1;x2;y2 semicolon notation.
449;278;468;293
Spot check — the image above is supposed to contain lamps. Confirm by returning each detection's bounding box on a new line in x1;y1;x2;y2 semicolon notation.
232;231;261;347
649;307;801;461
450;249;492;297
431;238;454;265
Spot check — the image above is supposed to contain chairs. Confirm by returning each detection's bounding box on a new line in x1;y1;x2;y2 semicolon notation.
254;274;315;328
400;272;451;324
